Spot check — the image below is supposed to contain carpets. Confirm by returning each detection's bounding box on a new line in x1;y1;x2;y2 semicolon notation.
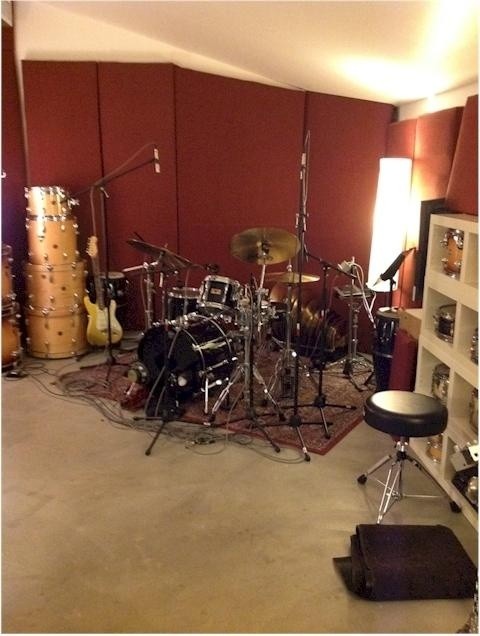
52;331;377;456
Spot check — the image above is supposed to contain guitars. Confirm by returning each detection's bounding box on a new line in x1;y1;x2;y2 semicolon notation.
84;236;124;347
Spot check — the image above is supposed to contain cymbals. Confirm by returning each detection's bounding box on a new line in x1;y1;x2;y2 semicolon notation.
230;227;300;266
261;272;320;284
267;284;351;361
126;238;192;269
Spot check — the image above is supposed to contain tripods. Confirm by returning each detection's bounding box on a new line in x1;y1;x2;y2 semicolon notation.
60;163;130;380
136;264;370;461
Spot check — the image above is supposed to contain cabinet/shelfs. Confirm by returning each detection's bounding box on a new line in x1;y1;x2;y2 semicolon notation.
410;214;478;533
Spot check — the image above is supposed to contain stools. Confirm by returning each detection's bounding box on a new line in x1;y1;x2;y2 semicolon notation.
355;390;462;524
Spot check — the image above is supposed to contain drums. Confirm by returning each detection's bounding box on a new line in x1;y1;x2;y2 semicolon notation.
197;274;241;317
431;363;449;405
2;246;24;370
23;184;89;359
137;312;238;403
89;272;128;308
433;303;455;343
467;388;477;432
425;435;442;463
168;286;200;318
371;307;398;390
440;229;464;274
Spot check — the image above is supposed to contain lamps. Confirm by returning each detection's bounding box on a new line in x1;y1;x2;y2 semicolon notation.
363;156;414;342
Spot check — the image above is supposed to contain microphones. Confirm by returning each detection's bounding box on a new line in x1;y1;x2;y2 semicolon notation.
153;147;161;175
